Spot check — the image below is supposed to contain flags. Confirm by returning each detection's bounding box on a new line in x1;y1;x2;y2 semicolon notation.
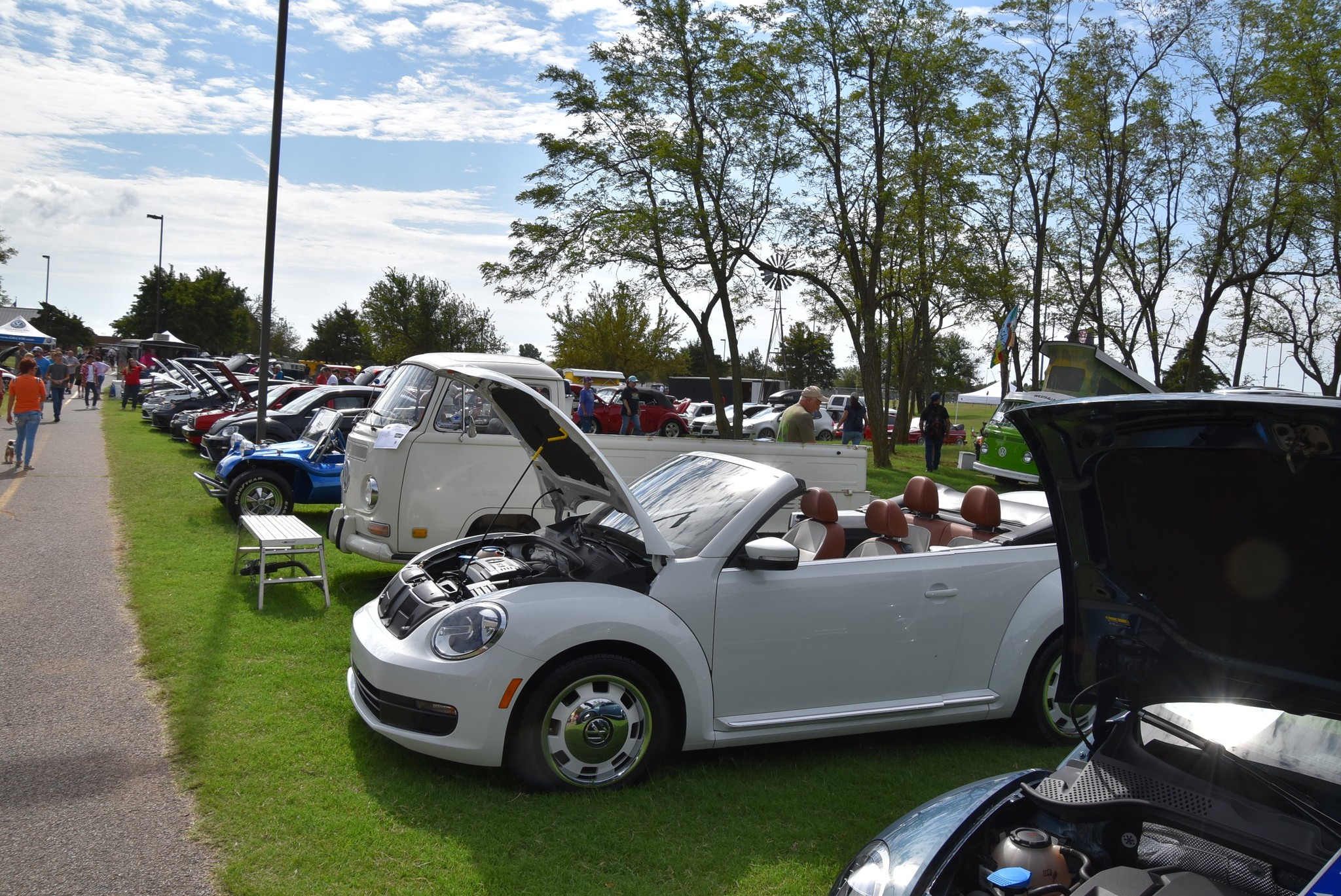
990;303;1018;369
10;301;16;307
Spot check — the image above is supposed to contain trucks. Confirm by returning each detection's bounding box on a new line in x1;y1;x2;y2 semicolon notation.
323;352;867;566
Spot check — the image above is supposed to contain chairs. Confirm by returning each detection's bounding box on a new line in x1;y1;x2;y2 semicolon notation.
781;486;846;562
485;417;510;435
846;499;914;558
971;427;980;443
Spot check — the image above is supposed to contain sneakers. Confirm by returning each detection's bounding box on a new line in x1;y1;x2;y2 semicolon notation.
120;407;136;410
66;387;102;401
55;417;60;422
925;470;933;473
15;464;35;470
86;406;101;410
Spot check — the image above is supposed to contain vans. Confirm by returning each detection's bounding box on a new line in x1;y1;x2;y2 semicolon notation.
974;342;1173;487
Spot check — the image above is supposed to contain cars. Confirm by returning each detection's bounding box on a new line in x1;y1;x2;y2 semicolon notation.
347;367;1116;796
820;389;1341;896
0;352;988;525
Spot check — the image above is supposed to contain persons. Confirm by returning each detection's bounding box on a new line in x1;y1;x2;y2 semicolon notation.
120;358;147;410
343;374;353;383
0;369;6;417
272;365;285;380
332;368;347;385
322;367;339;385
576;376;596;434
15;341;122;417
555;368;575;417
443;383;460;411
659;384;664;392
831;391;869;445
304;363;311;381
7;357;46;470
918;392;951;472
619;376;642;435
315;367;328;384
776;386;830;443
45;352;71;421
249;362;260;374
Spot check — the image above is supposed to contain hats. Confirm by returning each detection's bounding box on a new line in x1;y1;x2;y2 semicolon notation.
802;385;829;402
850;392;859;399
30;346;43;352
583;376;595;384
930;393;940;398
17;342;25;347
628;376;638;382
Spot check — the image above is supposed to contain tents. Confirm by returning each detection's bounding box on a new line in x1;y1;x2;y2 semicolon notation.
0;315;58;353
353;365;362;371
954;379;1018;426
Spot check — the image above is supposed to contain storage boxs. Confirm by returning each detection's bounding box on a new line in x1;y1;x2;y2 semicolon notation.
957;451;977;470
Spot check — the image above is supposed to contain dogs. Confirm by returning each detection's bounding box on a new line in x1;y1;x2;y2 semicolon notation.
4;439;17;464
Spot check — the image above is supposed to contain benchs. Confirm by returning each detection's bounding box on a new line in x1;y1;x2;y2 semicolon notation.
233;515;332;611
893;475;1002;553
